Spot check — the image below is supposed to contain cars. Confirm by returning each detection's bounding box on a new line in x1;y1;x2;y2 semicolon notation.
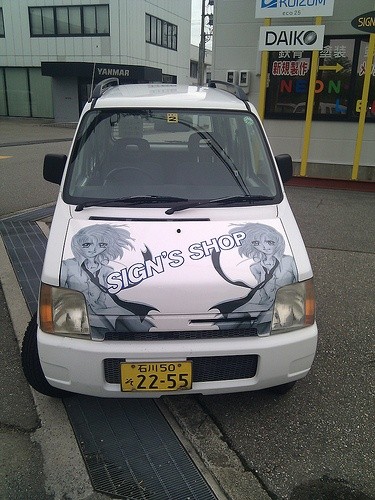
22;77;318;401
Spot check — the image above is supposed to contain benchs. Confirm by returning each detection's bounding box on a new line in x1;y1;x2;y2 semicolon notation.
111;144;226;183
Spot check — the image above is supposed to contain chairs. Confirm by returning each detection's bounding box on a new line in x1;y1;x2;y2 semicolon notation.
109;138;160;184
187;132;242;186
